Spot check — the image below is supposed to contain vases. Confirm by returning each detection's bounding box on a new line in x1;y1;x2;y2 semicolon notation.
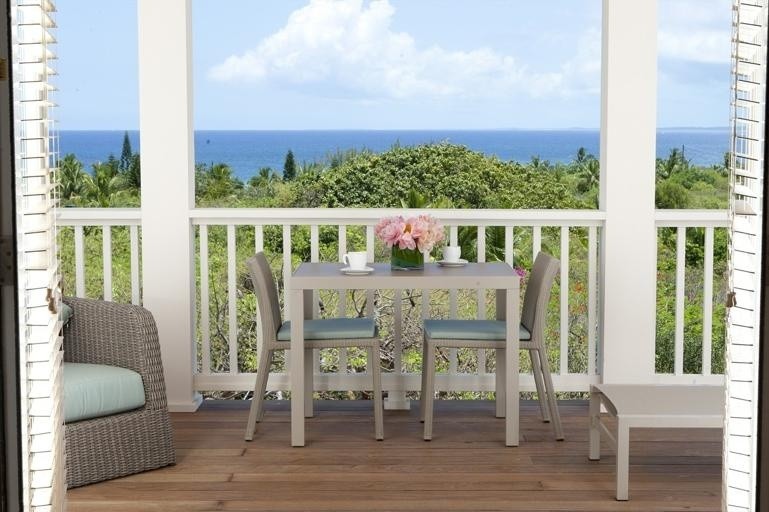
390;239;425;272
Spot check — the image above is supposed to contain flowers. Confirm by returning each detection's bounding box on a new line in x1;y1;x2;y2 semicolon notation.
374;212;445;268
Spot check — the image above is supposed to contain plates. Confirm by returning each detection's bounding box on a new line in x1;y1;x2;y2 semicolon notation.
340;267;374;275
436;260;468;266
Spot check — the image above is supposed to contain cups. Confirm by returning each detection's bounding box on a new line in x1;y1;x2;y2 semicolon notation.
343;252;367;268
443;246;461;261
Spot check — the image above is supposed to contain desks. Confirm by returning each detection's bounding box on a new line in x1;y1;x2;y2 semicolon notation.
286;260;523;448
587;384;726;502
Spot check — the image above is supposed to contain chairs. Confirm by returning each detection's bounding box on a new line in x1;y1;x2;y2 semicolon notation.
242;252;384;442
418;251;565;441
62;294;176;490
